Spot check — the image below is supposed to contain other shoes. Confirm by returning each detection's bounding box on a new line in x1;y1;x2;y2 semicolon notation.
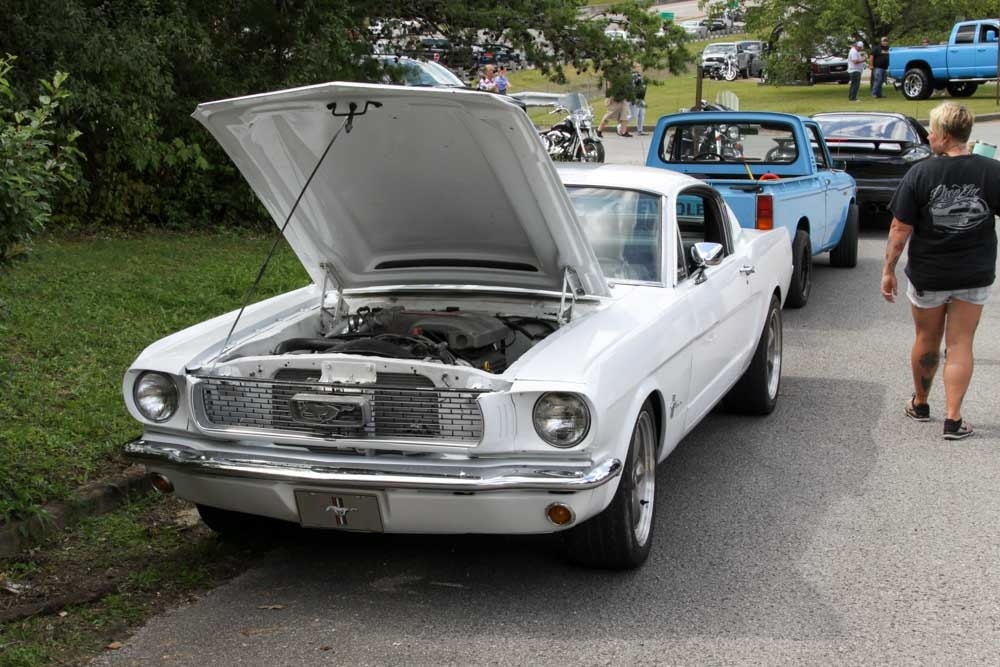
638;132;648;136
622;131;632;137
593;128;603;138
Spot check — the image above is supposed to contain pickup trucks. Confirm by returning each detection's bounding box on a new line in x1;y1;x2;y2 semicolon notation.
887;19;1000;104
624;111;859;308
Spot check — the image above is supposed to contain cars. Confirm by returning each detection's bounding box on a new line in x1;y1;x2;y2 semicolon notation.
782;110;932;229
800;47;849;84
606;16;727;45
116;77;794;571
355;54;469;89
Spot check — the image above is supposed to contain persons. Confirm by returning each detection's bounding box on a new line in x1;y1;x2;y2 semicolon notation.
476;64;509;94
869;37;890;98
921;38;929;44
847;41;865;101
596;62;649;137
881;103;1000;440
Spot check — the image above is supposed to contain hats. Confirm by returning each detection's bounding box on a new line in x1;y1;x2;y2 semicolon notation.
881;36;889;42
857;41;863;48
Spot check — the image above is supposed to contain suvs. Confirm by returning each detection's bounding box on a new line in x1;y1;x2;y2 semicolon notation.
701;42;751;81
416;37;523;71
735;40;766;78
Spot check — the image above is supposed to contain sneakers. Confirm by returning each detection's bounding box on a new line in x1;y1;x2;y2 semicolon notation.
943;416;973;439
904;391;931;421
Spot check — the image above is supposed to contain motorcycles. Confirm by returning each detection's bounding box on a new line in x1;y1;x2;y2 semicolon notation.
535;92;608;164
667;90;750;160
